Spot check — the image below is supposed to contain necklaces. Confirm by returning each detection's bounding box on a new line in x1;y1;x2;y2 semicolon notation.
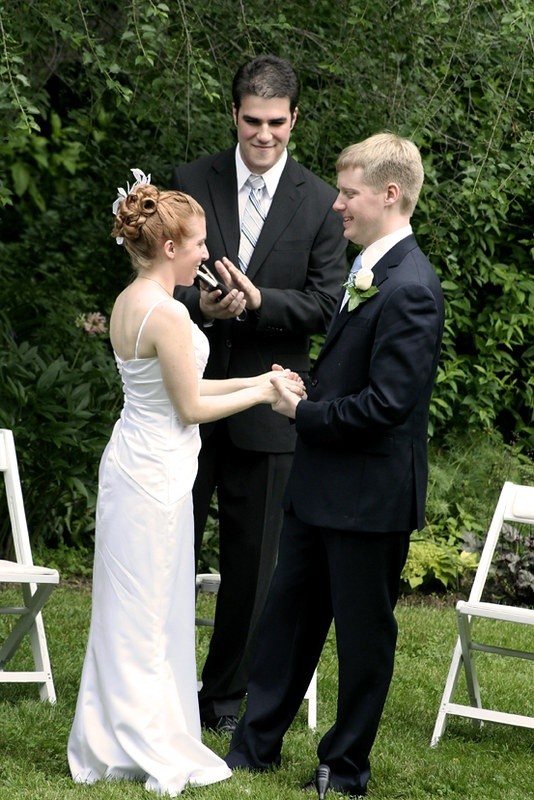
134;271;175;298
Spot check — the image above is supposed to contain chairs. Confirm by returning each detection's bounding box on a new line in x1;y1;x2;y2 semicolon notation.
0;428;59;704
194;574;317;733
429;481;534;746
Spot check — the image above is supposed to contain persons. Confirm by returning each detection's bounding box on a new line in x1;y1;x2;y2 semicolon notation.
222;133;446;799
171;57;350;731
67;169;307;798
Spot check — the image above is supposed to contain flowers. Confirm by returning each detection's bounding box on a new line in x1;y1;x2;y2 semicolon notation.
342;267;380;312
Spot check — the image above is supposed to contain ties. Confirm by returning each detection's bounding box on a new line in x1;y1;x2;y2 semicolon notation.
237;175;267;274
340;254;363;311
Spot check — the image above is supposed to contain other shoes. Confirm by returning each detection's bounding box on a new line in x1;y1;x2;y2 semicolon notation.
306;775;340;799
223;750;256;770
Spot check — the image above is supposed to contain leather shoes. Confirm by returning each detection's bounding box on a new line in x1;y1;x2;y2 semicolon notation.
212;714;238;733
